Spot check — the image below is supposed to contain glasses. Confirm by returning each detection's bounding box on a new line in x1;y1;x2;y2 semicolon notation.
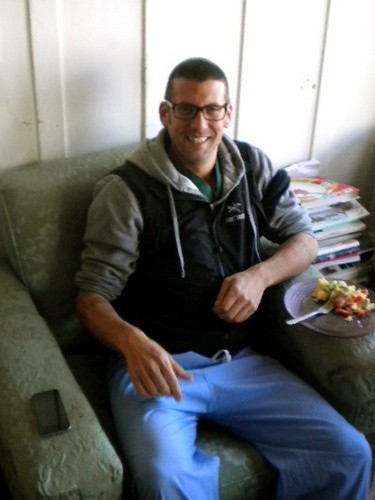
164;98;229;121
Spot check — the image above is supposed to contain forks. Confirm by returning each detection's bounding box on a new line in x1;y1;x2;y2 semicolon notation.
286;301;334;325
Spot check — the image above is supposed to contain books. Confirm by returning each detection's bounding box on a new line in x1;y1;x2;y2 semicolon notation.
287;176;371;270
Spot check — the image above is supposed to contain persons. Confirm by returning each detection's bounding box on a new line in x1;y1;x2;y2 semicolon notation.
74;57;373;500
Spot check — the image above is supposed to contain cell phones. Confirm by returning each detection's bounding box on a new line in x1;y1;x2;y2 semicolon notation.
30;389;73;439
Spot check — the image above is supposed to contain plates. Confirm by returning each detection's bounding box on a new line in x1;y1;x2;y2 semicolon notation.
283;278;375;337
